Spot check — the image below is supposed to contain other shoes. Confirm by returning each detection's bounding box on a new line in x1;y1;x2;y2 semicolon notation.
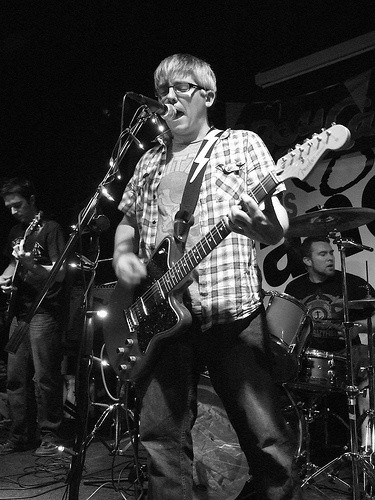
0;439;17;455
34;440;59;455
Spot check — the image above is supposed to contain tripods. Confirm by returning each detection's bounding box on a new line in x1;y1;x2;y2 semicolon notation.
294;237;375;500
80;373;143;455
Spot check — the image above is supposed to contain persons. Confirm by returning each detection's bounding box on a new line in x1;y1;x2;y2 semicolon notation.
283;235;375;324
112;53;300;500
0;177;62;455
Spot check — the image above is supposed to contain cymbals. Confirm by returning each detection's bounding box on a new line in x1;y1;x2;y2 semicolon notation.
282;207;375;239
331;300;375;309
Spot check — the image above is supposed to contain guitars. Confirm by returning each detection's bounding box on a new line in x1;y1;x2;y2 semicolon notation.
99;122;354;380
1;211;48;333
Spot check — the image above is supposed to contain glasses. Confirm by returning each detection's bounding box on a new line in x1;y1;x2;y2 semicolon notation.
154;82;206;98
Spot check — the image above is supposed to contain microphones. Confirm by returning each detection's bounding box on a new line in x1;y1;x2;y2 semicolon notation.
128;90;176;122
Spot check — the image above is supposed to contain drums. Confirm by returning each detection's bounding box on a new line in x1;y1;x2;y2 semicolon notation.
178;374;309;500
252;289;310;362
284;349;348;394
303;316;346;348
